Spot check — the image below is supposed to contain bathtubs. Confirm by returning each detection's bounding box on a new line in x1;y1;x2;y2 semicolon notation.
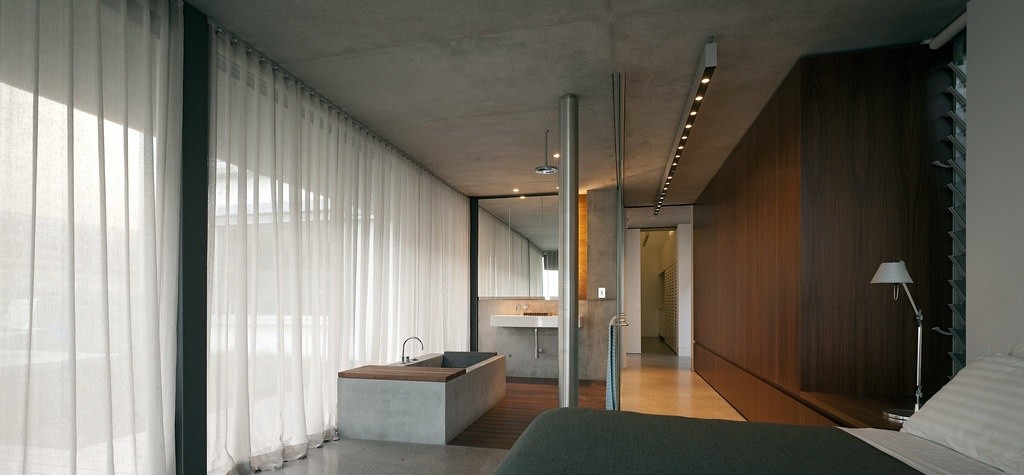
336;351;508;445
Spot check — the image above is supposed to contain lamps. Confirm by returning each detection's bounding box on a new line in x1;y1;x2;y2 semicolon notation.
869;260;925;420
534;130;558;175
653;35;717;215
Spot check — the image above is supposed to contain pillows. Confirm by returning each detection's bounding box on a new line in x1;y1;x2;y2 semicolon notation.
901;349;1024;475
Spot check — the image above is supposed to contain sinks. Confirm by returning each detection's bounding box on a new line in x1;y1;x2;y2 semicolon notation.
490;314;584;328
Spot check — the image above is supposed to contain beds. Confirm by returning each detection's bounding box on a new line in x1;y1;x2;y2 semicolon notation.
493;407;1008;475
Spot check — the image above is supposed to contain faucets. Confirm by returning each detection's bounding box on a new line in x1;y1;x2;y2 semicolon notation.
400;336;424;364
515;305;520;311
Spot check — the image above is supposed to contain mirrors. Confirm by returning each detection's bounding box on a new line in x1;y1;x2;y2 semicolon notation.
477;194;560;300
